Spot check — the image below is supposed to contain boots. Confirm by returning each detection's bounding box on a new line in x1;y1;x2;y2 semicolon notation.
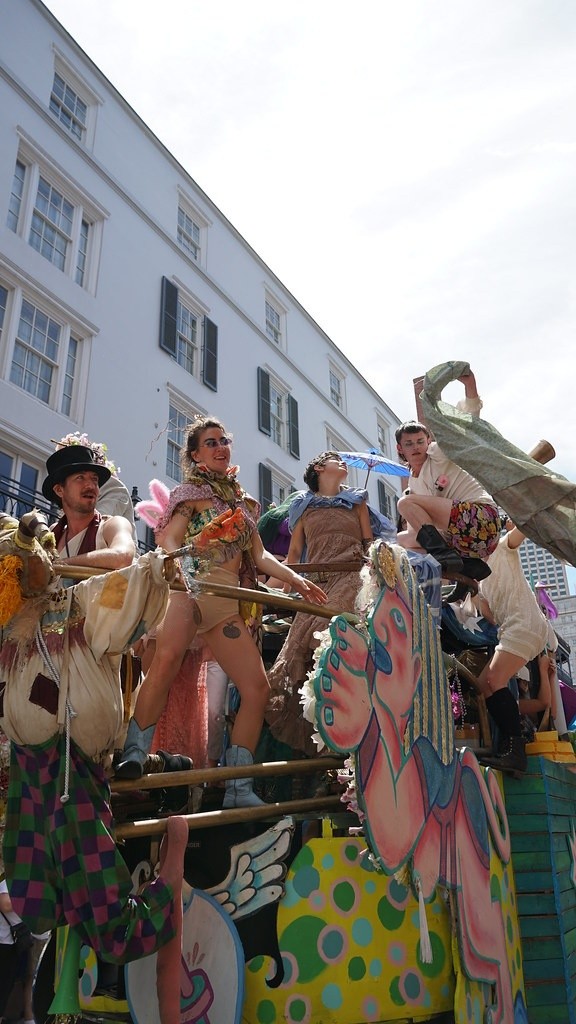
222;745;265;808
113;715;156;778
416;524;464;572
480;736;528;780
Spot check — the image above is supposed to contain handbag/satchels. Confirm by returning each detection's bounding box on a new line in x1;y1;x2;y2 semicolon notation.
1;911;34;950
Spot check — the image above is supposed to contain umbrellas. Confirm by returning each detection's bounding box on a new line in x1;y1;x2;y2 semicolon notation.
338;448;410;489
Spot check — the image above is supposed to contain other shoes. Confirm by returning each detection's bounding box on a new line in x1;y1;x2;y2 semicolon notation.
155;748;194;817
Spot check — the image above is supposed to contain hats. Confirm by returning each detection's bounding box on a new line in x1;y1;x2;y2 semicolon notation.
514;665;530;681
41;445;112;502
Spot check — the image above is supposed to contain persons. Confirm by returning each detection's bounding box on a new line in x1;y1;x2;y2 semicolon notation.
0;369;576;1024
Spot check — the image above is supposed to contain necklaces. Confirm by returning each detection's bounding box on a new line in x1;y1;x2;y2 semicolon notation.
65;529;77;558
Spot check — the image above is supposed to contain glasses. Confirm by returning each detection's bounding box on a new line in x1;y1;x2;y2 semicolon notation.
199;437;232;448
